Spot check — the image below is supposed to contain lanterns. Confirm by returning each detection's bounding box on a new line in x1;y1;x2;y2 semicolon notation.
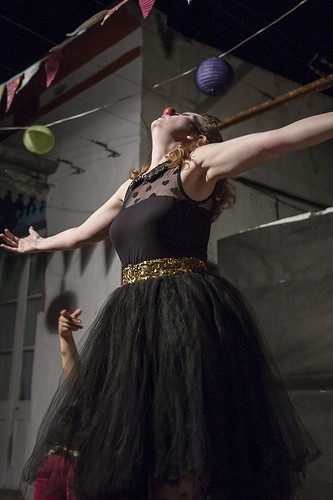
20;124;53;154
196;57;234;96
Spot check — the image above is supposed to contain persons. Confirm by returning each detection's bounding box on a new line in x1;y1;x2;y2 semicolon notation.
57;308;81;379
2;110;332;498
